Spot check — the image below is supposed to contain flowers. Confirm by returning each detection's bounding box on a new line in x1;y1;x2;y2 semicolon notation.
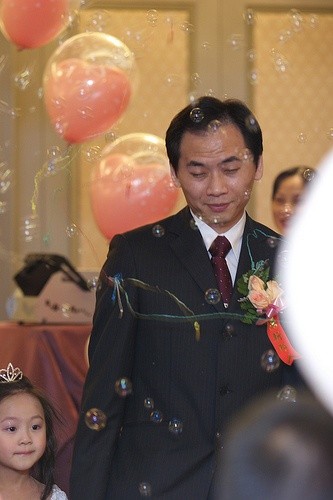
234;259;286;326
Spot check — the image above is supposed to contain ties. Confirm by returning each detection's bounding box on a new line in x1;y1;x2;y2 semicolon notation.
207;235;233;309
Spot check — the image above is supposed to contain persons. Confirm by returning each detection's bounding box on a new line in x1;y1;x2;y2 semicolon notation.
0;370;68;500
273;165;320;234
68;96;306;500
216;393;333;500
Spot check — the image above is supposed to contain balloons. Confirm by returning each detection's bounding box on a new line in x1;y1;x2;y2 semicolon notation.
42;33;141;147
0;0;85;51
90;135;180;241
